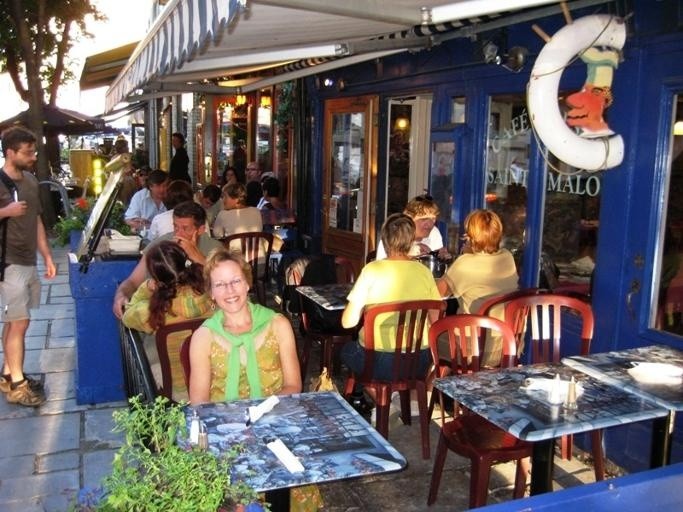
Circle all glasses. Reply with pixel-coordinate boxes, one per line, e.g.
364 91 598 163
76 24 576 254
246 168 258 171
14 149 38 156
210 275 246 290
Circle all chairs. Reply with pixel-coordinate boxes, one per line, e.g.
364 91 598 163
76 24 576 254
153 215 593 462
502 298 605 494
426 315 535 512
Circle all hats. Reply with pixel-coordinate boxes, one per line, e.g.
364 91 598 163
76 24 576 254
579 47 619 89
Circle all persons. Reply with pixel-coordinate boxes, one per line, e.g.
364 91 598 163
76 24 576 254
340 194 528 424
0 126 56 407
113 133 302 390
188 247 301 512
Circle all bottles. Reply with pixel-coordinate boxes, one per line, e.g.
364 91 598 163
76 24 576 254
564 376 578 410
549 372 562 405
198 420 209 449
189 409 199 443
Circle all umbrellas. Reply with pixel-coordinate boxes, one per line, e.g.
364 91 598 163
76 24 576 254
0 105 106 175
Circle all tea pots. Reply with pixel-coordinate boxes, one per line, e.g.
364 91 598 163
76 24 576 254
417 251 447 278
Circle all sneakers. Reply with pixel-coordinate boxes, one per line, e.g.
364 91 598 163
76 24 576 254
0 373 47 406
